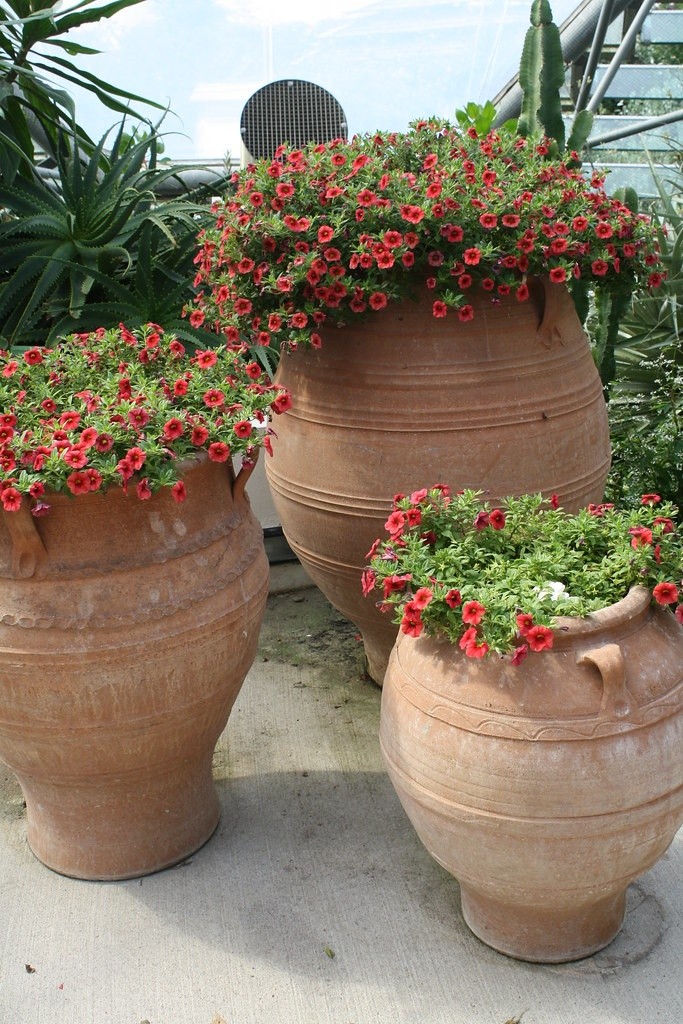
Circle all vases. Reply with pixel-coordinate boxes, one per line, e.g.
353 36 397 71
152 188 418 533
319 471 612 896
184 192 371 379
265 273 613 687
0 446 270 881
378 583 683 964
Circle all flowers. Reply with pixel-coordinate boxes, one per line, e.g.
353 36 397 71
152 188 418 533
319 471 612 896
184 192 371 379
181 117 668 356
0 321 293 519
361 479 683 669
